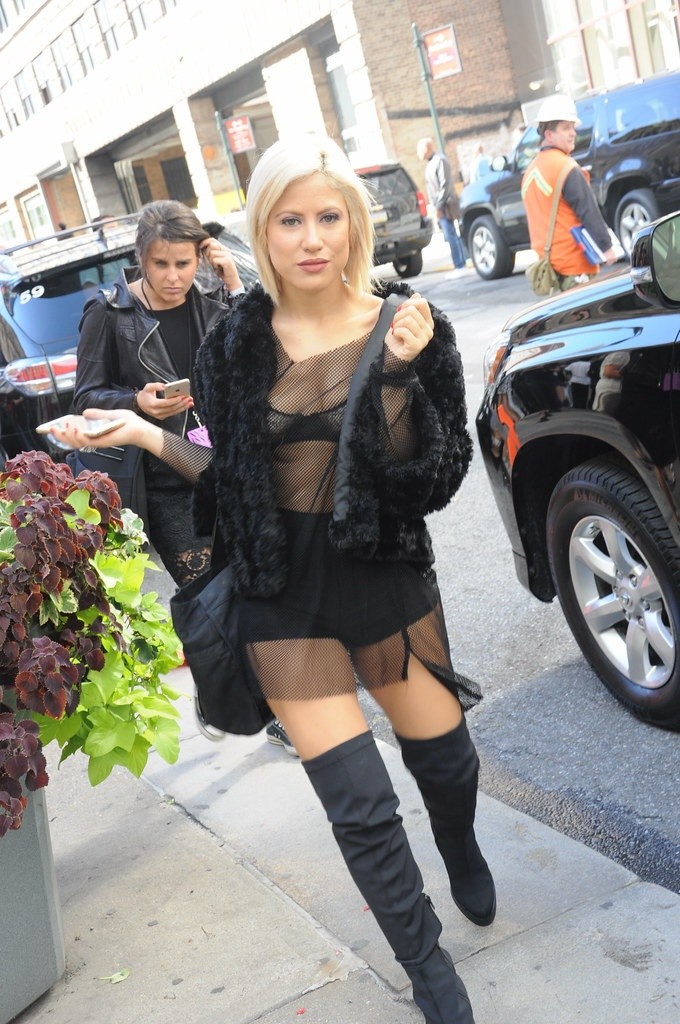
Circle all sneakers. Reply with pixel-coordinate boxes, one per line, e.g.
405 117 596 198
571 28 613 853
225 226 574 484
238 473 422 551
267 719 299 757
194 684 225 742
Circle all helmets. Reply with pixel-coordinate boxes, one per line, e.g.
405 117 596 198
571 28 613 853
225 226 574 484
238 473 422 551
537 94 582 129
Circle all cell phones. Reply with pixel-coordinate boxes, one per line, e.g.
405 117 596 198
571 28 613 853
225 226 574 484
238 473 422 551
164 378 190 400
35 414 126 439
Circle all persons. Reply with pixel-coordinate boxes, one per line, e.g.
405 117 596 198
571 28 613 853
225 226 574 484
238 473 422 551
91 215 118 234
416 138 469 281
57 223 72 241
37 134 498 1024
72 199 299 757
520 94 619 294
474 146 492 180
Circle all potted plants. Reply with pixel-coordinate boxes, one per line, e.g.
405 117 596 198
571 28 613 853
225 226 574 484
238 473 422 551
0 447 189 1024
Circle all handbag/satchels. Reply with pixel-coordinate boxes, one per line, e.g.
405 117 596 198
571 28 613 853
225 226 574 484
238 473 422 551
525 258 559 296
66 290 150 551
169 559 274 735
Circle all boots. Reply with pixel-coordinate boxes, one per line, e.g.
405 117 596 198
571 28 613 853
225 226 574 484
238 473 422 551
301 729 474 1024
393 705 497 927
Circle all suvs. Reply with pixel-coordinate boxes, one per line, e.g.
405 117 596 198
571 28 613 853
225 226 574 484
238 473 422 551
454 72 680 279
0 209 263 480
475 211 680 733
356 159 435 280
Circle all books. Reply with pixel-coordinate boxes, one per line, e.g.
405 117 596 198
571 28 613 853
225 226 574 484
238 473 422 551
581 226 626 262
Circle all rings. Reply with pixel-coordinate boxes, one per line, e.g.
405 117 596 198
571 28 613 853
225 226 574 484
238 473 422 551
79 445 98 453
